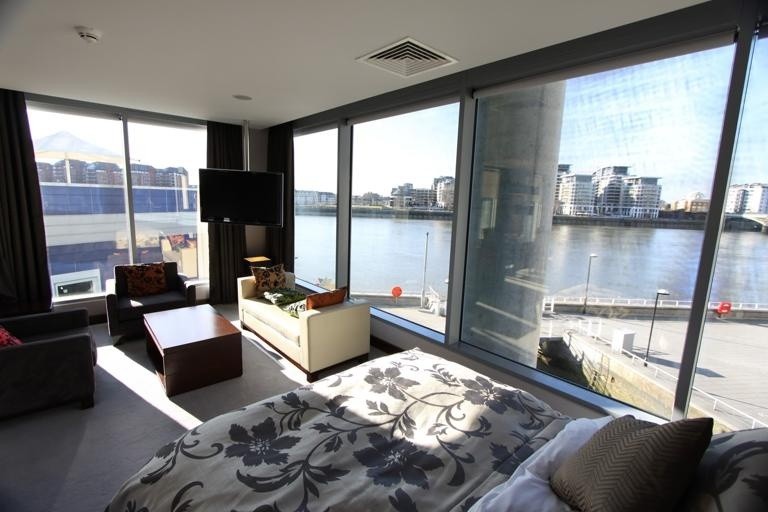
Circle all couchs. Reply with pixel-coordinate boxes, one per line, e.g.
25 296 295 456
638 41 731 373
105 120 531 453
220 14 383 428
236 272 371 382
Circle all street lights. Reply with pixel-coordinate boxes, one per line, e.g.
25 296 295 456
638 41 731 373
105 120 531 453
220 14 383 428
643 289 671 367
582 253 599 315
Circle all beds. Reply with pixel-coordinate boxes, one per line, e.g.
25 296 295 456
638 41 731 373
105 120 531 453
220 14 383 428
107 347 767 511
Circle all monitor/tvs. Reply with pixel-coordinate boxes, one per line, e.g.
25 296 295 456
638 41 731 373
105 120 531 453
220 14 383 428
199 169 284 228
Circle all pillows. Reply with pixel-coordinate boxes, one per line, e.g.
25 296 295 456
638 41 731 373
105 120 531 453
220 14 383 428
121 263 168 296
549 418 715 511
251 264 346 317
1 325 23 349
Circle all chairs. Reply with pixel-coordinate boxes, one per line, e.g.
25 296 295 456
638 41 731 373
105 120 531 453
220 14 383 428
106 263 198 347
0 308 97 420
